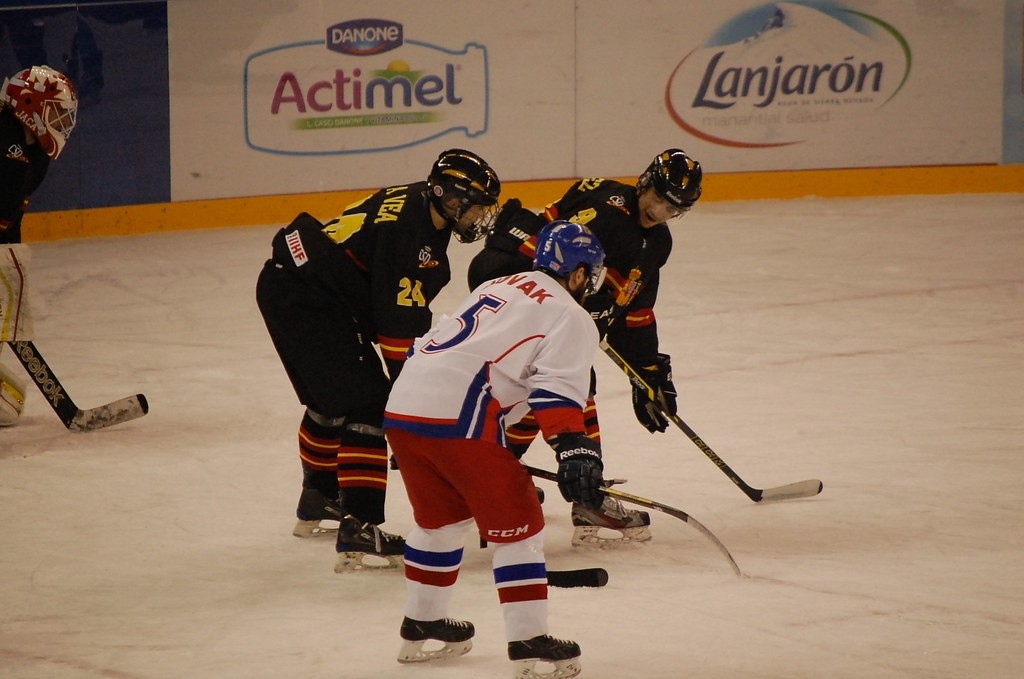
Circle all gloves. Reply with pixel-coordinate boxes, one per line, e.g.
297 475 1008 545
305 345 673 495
624 353 678 433
554 437 607 510
484 195 547 251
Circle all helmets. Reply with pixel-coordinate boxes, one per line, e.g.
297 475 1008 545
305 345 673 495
2 64 78 160
428 148 501 245
636 148 704 220
534 219 606 298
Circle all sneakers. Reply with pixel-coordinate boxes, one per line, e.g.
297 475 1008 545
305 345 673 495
333 517 407 574
571 495 652 549
292 486 341 538
397 616 475 663
508 634 583 679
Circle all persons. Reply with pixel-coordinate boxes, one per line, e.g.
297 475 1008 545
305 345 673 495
467 148 704 548
0 65 77 427
383 220 608 679
255 149 500 577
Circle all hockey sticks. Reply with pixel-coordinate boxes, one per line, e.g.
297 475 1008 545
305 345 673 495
7 340 150 433
521 464 744 579
600 340 824 505
